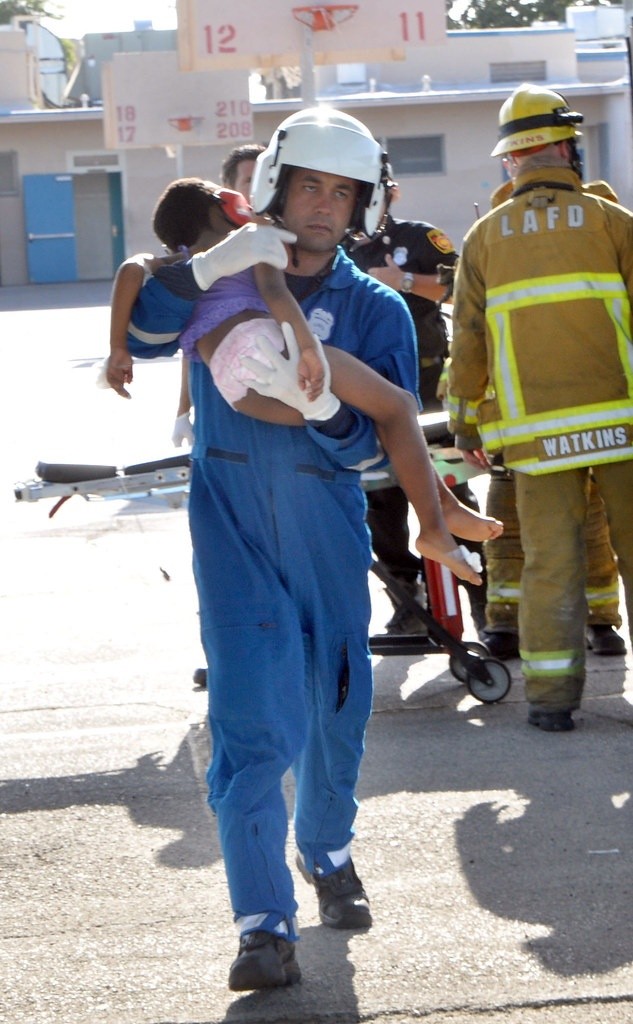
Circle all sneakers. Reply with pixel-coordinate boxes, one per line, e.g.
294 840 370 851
229 928 300 993
294 849 373 930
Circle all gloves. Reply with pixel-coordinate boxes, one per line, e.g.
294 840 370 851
239 322 341 422
191 221 296 292
170 412 194 448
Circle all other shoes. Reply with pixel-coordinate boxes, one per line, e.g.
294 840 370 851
526 700 575 732
374 630 429 658
480 633 519 662
585 621 628 656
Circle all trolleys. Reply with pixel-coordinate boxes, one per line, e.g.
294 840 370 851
14 411 513 704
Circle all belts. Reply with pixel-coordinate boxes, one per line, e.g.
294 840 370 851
419 355 444 370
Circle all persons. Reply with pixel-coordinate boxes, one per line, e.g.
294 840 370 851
172 143 627 657
449 84 633 735
107 179 504 584
125 109 422 992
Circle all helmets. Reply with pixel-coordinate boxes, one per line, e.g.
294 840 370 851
249 107 394 241
488 83 584 158
